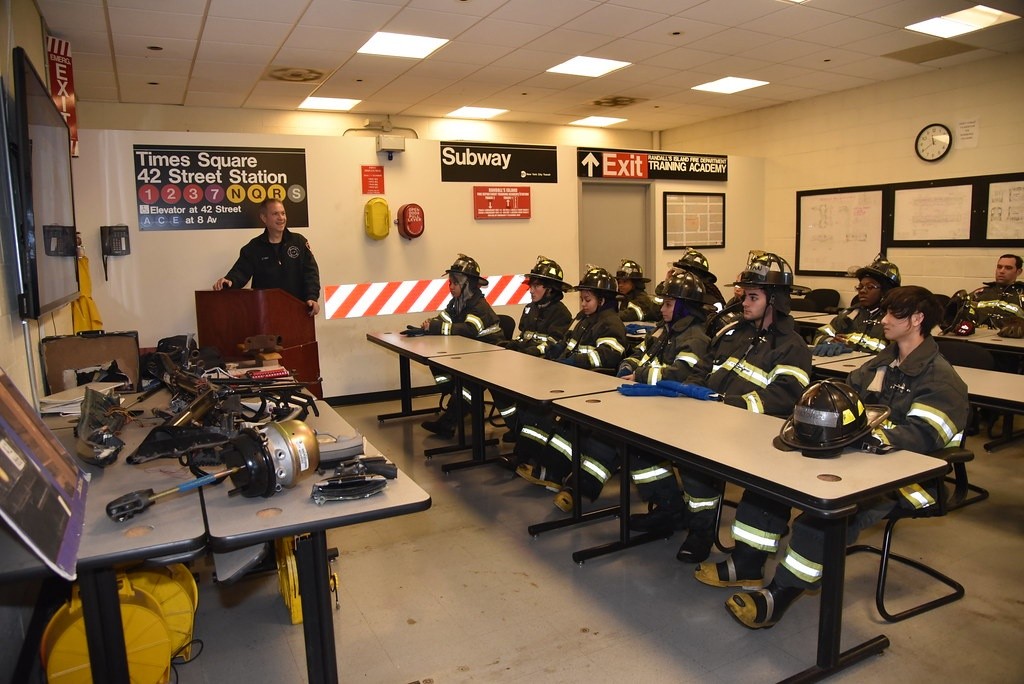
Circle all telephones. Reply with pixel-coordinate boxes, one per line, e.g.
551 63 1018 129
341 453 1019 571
100 226 130 256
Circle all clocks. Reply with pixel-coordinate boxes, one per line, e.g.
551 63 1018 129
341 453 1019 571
914 123 952 162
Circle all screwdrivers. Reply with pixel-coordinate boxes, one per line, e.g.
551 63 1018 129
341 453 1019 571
124 386 161 409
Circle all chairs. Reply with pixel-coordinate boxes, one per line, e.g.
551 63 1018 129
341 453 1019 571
845 410 989 622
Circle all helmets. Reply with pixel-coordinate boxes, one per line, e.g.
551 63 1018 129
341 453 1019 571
524 256 574 293
724 252 812 296
655 271 711 305
673 247 718 284
572 264 623 298
779 379 892 451
938 289 978 337
613 259 651 283
445 254 489 286
855 260 901 290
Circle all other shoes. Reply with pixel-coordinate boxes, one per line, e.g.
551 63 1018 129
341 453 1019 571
515 462 562 493
420 406 466 440
677 509 717 564
725 562 810 630
552 486 574 513
628 497 689 533
693 541 769 591
502 428 517 443
496 452 534 471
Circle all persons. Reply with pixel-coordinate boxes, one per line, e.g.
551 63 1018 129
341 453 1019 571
611 267 713 502
497 268 626 476
212 198 320 317
421 253 504 439
488 257 575 443
812 253 902 360
693 285 969 630
615 248 812 563
963 254 1024 437
515 267 629 513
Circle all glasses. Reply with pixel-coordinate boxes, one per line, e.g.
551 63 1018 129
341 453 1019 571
854 284 884 292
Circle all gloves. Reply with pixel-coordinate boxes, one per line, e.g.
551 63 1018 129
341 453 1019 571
617 383 684 398
399 324 427 337
810 343 853 357
656 378 723 402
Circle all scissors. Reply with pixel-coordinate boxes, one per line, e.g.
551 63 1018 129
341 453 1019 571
184 350 206 369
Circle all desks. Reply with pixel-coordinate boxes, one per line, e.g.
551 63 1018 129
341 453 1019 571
0 289 1024 684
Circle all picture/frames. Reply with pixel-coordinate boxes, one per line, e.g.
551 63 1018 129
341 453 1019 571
663 192 726 248
795 172 1024 276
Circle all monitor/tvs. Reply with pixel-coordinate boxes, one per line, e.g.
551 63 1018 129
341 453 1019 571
10 46 83 321
